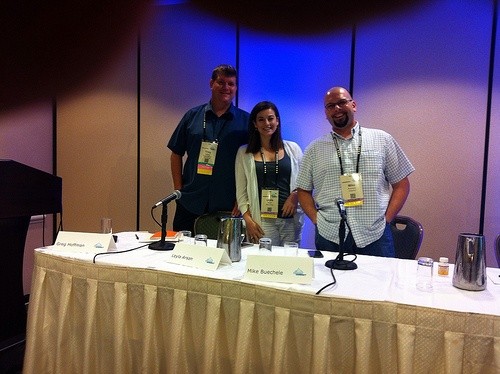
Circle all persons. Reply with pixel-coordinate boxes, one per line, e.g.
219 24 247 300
295 86 416 257
165 64 251 237
235 101 304 248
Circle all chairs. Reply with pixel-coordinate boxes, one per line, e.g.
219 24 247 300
194 211 248 242
390 215 424 260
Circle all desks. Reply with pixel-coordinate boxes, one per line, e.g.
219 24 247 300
24 231 500 374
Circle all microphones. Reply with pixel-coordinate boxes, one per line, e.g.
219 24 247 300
152 190 182 208
335 196 346 212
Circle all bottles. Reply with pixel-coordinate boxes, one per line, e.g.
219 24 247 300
438 257 449 278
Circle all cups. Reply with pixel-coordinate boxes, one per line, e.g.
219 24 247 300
284 242 298 257
259 238 271 251
416 257 433 290
194 234 207 247
179 231 191 242
100 218 112 234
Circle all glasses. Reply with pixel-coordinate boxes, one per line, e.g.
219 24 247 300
325 98 352 112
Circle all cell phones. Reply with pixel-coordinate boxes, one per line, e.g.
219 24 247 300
308 250 323 258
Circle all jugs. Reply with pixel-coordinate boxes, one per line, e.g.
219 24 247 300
453 232 487 290
217 218 241 262
